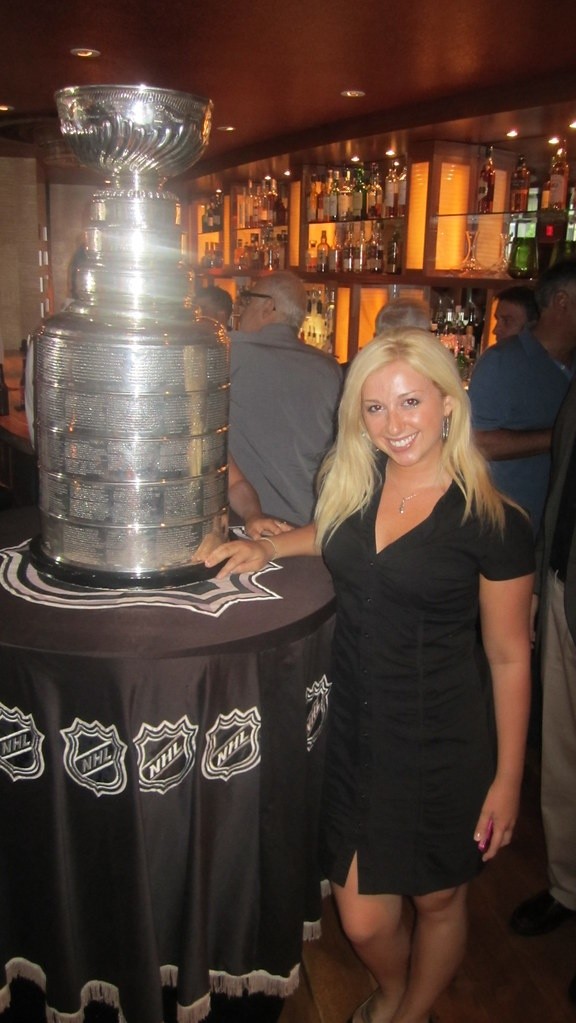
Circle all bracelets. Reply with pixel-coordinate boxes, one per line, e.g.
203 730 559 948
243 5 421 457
260 538 276 560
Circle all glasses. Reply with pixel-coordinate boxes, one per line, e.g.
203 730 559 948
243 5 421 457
239 289 276 312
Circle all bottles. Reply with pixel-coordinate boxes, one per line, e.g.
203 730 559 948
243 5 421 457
475 138 576 282
300 158 410 277
189 178 290 272
296 286 336 355
429 287 487 386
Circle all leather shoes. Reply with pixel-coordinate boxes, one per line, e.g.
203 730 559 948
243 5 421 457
509 888 576 938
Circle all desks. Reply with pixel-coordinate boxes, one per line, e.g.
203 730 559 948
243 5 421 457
0 504 335 1023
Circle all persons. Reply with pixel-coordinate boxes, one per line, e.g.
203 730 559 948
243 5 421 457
508 373 576 1010
493 287 538 342
470 255 576 770
372 298 432 336
205 326 535 1022
23 248 295 538
192 288 233 331
226 272 345 525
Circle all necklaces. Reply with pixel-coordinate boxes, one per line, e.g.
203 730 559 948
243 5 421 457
399 493 418 514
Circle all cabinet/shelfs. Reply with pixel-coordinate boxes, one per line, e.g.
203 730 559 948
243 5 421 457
166 66 576 391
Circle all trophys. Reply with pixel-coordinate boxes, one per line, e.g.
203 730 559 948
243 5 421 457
33 85 228 588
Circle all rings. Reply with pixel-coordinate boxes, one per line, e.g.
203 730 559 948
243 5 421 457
278 521 286 526
